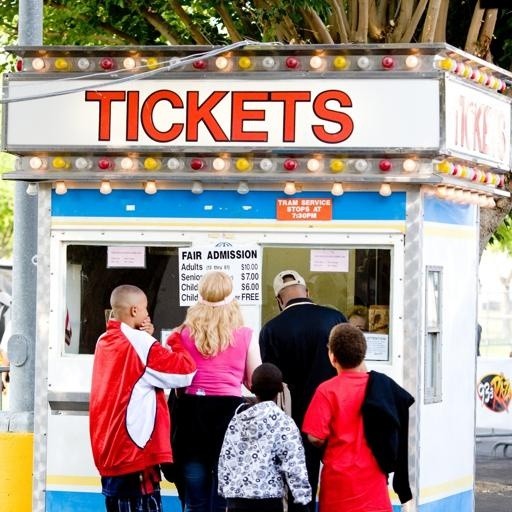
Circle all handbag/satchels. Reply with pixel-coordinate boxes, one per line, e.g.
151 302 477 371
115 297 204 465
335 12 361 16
160 389 178 483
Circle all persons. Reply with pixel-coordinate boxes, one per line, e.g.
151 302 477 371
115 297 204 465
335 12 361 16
216 362 314 512
258 270 349 511
345 304 369 334
168 270 258 512
93 284 198 511
299 322 395 510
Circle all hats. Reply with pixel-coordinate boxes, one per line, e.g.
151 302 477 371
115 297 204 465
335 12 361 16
274 270 306 311
